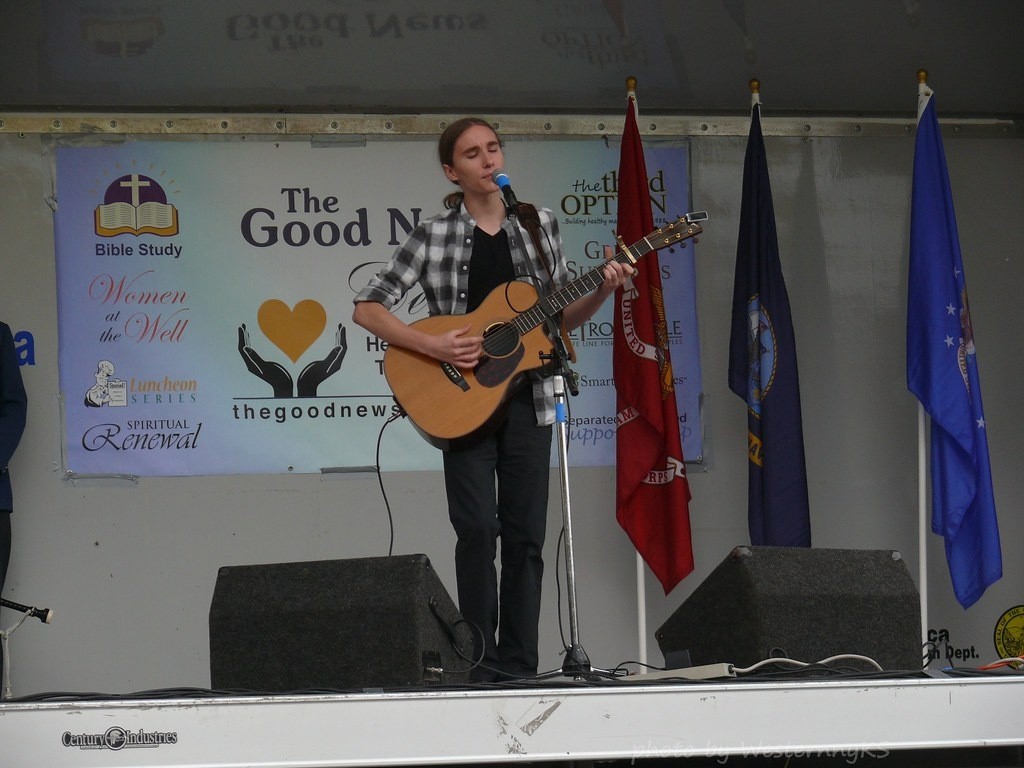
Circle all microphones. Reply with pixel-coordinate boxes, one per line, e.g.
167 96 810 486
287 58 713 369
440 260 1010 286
491 167 520 217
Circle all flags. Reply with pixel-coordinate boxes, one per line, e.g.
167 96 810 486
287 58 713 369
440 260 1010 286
907 91 1004 610
729 102 812 548
614 98 695 596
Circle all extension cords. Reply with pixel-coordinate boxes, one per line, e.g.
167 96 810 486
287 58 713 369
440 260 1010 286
614 663 737 681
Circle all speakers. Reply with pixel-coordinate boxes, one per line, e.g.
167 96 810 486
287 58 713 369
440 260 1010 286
210 553 473 689
654 545 921 681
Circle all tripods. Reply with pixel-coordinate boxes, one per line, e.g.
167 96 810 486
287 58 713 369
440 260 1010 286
505 202 627 683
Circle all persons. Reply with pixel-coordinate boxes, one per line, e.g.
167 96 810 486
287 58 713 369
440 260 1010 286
351 117 634 685
0 319 28 698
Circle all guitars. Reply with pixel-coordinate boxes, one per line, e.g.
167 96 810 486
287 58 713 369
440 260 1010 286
383 210 709 453
